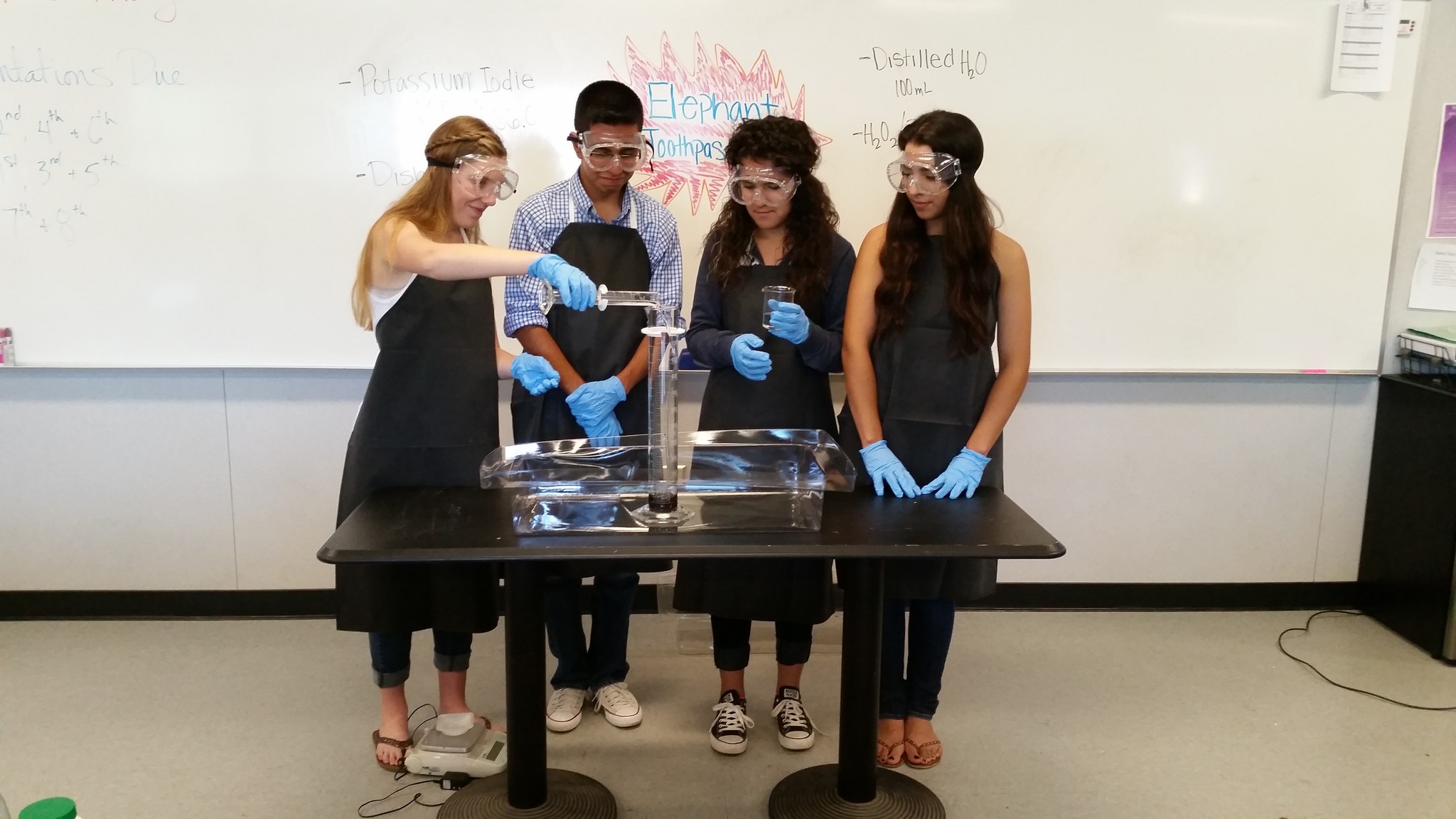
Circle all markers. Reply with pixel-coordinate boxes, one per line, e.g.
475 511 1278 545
0 328 12 363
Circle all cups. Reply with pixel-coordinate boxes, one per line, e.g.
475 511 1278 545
761 285 796 331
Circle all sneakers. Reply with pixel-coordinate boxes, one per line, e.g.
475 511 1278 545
546 687 588 731
593 681 642 728
772 685 829 750
709 688 754 754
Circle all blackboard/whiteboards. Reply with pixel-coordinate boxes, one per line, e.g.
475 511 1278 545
0 0 1430 377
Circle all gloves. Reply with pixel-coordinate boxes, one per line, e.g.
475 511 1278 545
511 352 561 396
767 299 810 345
565 376 626 426
859 440 921 498
921 446 992 500
527 253 597 311
583 411 623 453
729 333 772 380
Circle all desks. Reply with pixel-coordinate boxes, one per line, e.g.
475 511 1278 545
1354 372 1456 667
315 484 1066 819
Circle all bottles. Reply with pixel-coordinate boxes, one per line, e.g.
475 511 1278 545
18 796 82 819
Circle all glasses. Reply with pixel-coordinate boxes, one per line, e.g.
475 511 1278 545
453 154 519 200
577 131 647 171
727 164 801 207
887 152 960 195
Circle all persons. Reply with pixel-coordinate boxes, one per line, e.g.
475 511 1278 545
840 113 1032 766
670 117 857 757
333 115 595 771
505 81 682 730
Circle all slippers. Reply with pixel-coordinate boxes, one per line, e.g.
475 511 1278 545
480 716 507 734
877 738 906 768
372 729 414 773
904 737 941 769
